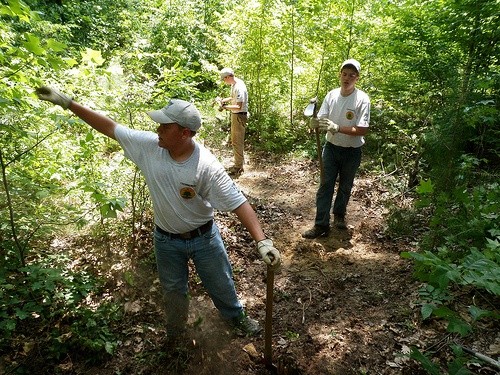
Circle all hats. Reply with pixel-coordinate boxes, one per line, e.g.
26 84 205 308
146 100 202 131
218 68 234 80
341 59 362 74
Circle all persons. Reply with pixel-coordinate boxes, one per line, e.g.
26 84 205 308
217 66 248 175
36 85 280 352
301 57 370 240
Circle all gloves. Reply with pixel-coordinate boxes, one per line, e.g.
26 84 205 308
35 84 73 110
310 116 319 129
319 118 339 135
256 239 282 271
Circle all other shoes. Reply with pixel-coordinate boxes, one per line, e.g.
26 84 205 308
302 225 331 239
158 335 184 369
234 315 263 336
334 216 348 230
225 166 243 175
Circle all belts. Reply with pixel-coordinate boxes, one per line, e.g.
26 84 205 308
155 220 213 240
233 112 247 114
